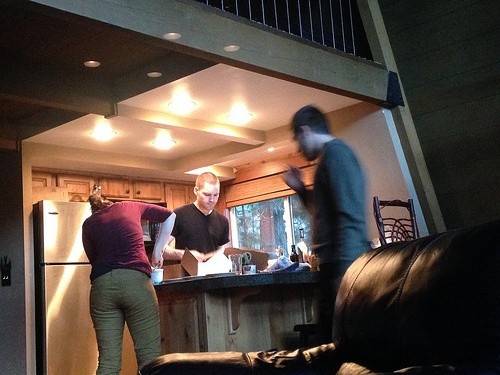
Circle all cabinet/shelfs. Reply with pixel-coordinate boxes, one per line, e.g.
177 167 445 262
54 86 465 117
98 174 166 204
32 171 98 202
164 179 217 213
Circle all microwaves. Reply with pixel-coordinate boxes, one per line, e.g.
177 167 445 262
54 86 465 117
140 219 162 253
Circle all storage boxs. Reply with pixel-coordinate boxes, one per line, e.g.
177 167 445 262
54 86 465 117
161 247 232 280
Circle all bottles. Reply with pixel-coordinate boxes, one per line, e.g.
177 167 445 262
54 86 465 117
276 246 284 258
290 245 299 263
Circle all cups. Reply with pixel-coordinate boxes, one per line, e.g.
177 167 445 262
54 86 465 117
228 254 243 275
243 264 256 274
152 268 164 284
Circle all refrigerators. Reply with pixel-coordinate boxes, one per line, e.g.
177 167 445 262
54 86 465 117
33 199 138 375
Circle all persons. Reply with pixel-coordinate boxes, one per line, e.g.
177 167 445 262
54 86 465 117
162 172 231 266
283 104 370 345
82 193 176 375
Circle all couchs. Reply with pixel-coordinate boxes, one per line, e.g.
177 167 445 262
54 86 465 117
137 218 500 375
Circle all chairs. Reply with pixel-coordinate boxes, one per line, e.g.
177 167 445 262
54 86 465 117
293 195 420 347
